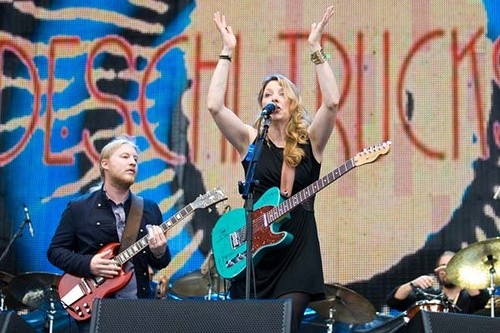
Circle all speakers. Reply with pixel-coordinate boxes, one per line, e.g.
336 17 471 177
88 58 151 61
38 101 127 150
392 309 500 332
89 296 297 333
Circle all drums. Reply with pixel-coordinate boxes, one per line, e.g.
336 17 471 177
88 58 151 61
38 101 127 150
406 299 450 320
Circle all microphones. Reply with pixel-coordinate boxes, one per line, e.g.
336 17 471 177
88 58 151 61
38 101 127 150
23 205 37 237
262 102 277 116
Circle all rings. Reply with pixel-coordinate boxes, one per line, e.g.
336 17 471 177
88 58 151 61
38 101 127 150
153 242 156 243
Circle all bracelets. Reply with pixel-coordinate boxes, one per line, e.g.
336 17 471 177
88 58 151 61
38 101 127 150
311 49 327 64
219 55 231 62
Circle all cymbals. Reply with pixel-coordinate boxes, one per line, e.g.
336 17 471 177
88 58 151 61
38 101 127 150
9 271 64 312
308 283 376 324
444 237 500 289
172 268 232 297
0 270 15 301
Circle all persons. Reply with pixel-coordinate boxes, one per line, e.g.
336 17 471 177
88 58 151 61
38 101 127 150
387 250 500 317
206 4 340 333
47 140 171 333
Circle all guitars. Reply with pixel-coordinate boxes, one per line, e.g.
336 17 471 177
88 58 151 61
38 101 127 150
211 139 392 280
58 184 227 322
